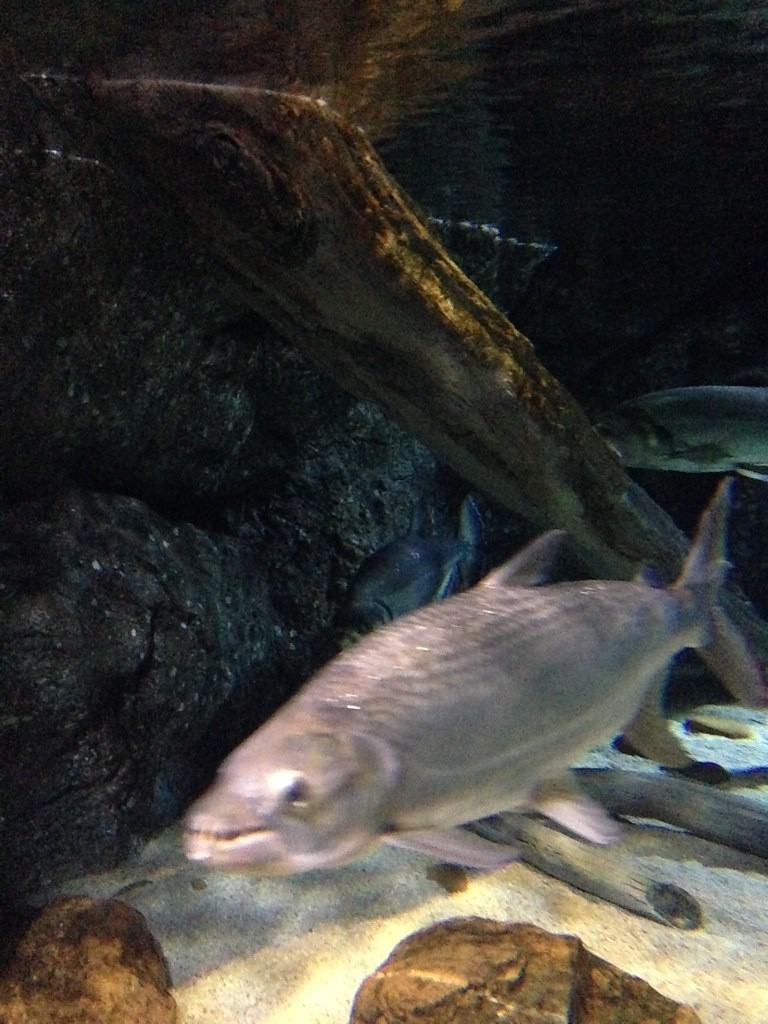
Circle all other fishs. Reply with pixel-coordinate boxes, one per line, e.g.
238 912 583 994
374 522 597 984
175 476 768 880
594 385 768 484
